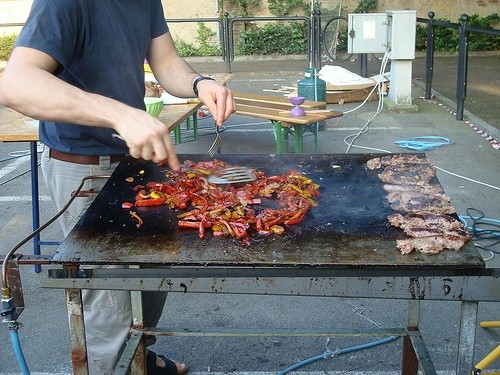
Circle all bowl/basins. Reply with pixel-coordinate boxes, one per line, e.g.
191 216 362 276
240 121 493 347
143 97 163 117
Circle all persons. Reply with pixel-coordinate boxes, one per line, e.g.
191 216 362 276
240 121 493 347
0 0 236 375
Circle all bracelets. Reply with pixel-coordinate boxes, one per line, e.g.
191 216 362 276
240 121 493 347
193 77 214 95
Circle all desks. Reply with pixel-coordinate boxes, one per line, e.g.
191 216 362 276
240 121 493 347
0 72 236 273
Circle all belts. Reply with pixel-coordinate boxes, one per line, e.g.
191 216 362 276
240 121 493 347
50 148 125 165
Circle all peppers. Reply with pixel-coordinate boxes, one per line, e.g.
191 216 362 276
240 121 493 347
123 159 320 239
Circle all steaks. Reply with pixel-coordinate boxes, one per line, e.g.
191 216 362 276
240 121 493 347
366 154 473 255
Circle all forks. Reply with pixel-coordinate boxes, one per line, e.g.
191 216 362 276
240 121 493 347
208 123 221 157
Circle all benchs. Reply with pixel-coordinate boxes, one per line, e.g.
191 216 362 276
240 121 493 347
173 91 344 154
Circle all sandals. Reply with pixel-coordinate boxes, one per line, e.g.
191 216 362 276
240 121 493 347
146 349 188 375
